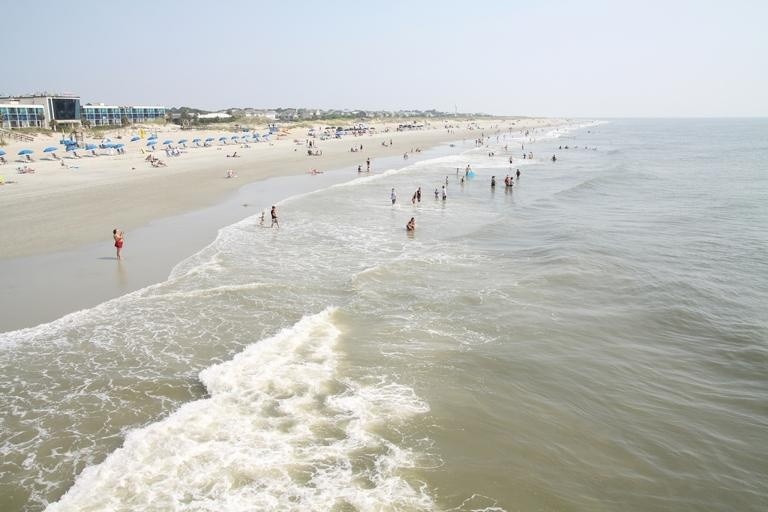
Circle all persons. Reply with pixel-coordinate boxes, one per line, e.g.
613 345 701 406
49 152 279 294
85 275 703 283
114 228 123 255
0 118 597 233
